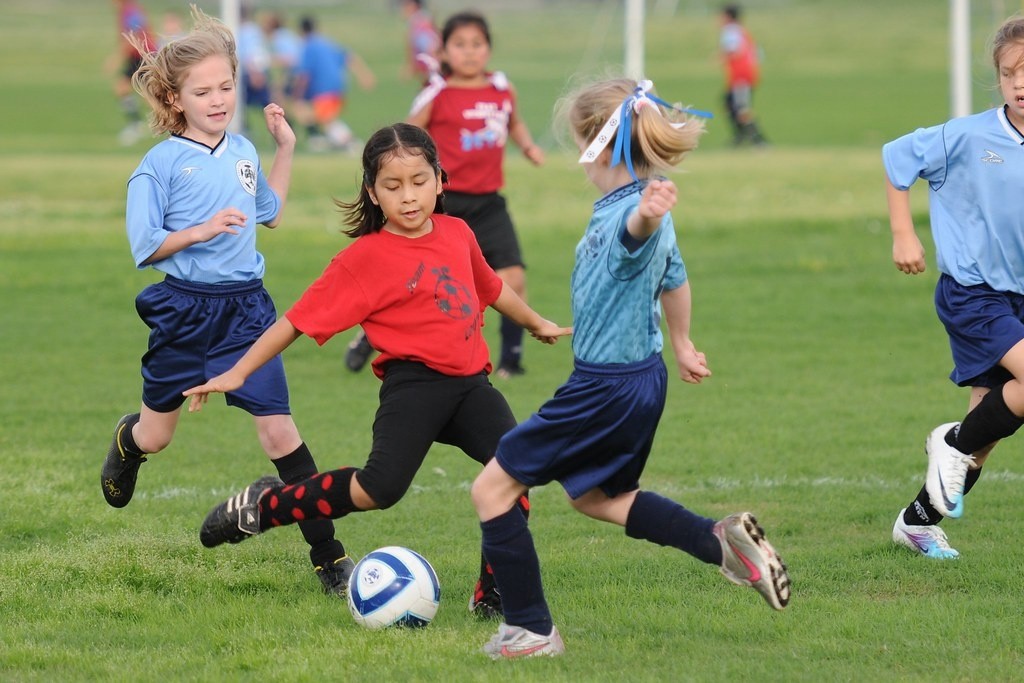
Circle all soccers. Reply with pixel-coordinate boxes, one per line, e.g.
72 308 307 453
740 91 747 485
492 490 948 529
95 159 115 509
347 545 441 630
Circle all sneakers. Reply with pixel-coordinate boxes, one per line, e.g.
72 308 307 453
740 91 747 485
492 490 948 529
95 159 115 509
496 361 526 378
100 413 145 508
468 622 565 662
309 541 354 599
713 513 793 612
469 580 503 617
347 329 374 371
925 423 978 521
891 508 960 561
199 476 284 548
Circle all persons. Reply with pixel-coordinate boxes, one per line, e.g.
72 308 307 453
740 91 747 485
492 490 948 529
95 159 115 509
239 2 376 154
720 6 766 143
100 16 357 599
882 15 1024 560
471 78 792 659
400 0 446 89
347 12 544 379
182 123 574 619
107 0 186 143
